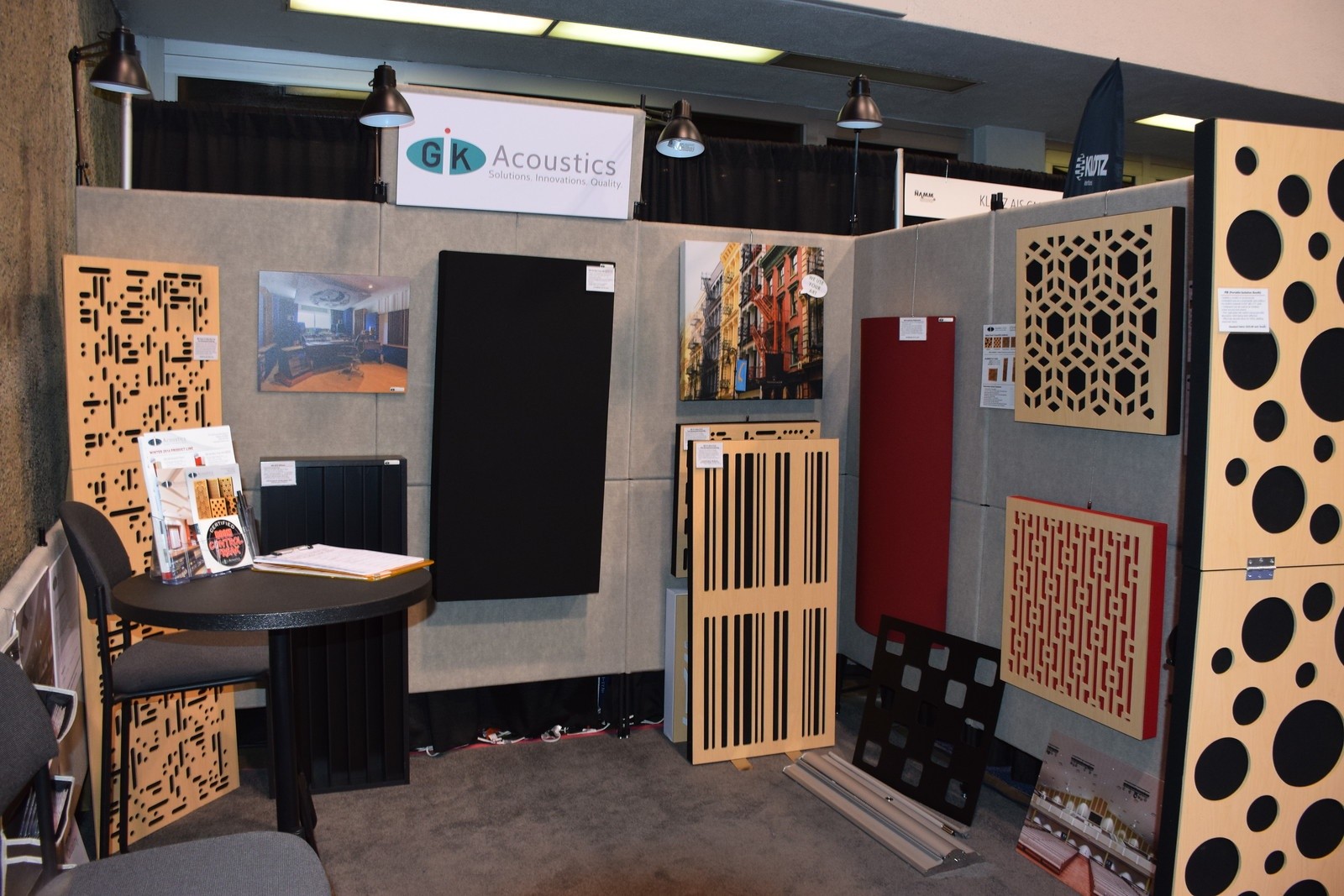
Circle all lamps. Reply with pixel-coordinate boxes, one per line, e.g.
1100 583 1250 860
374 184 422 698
66 23 153 188
783 749 984 876
359 60 414 205
642 94 706 160
836 74 882 238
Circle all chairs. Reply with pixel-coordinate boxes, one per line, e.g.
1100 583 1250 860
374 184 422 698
336 334 372 378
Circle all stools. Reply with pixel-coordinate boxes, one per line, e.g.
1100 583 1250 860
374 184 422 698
58 498 321 864
0 650 332 896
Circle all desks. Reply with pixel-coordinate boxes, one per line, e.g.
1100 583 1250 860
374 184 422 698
110 560 431 847
